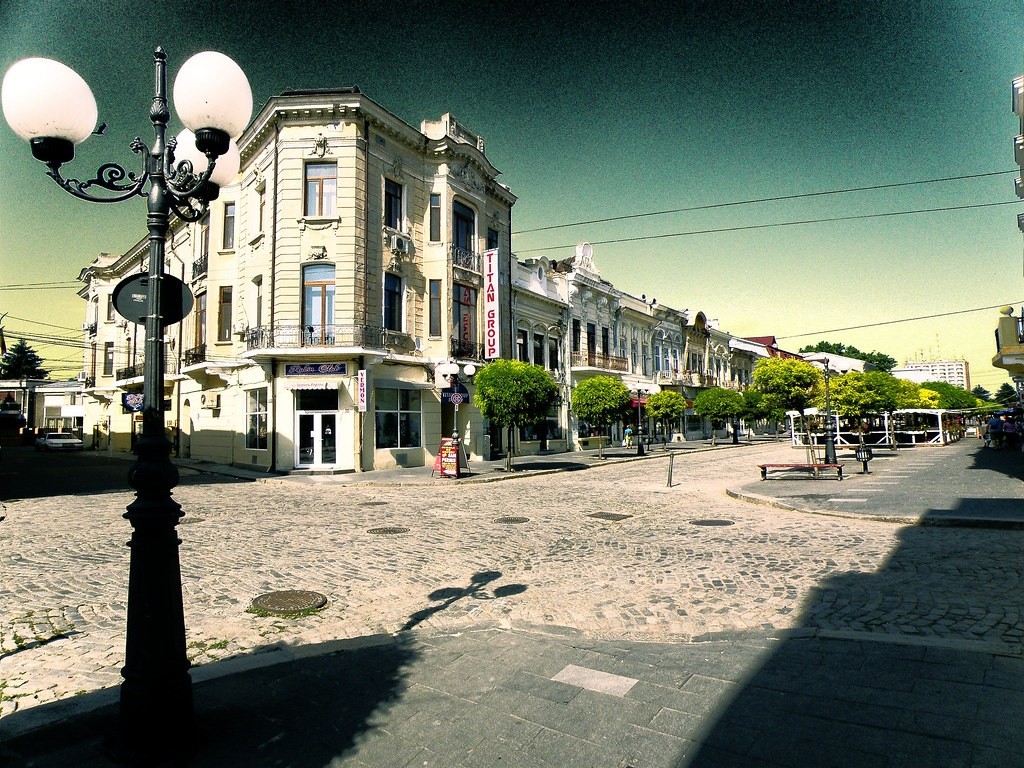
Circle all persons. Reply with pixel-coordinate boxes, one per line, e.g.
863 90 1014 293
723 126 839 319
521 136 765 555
624 425 634 450
959 413 1024 454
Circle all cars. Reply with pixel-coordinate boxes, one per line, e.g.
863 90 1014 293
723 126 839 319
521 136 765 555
36 432 85 452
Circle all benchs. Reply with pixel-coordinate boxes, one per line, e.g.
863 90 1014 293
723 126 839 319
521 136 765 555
759 463 845 481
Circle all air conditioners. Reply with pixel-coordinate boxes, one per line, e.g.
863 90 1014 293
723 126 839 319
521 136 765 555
391 235 409 254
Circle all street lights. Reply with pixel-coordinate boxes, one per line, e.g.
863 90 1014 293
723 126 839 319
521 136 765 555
628 379 659 456
0 43 258 768
823 355 839 463
434 361 476 478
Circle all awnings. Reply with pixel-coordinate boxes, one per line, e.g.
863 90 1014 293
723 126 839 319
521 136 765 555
629 394 646 408
372 377 436 391
287 379 343 390
